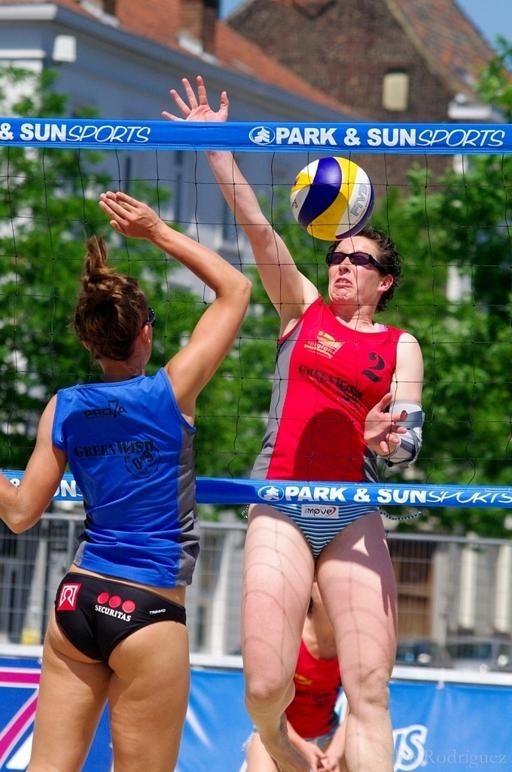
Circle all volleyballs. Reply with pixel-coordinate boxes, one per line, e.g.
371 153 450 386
290 157 374 241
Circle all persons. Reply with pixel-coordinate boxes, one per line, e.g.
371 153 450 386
163 76 423 772
245 563 352 772
0 191 252 772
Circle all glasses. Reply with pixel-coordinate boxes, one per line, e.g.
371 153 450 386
142 307 156 333
325 252 387 277
